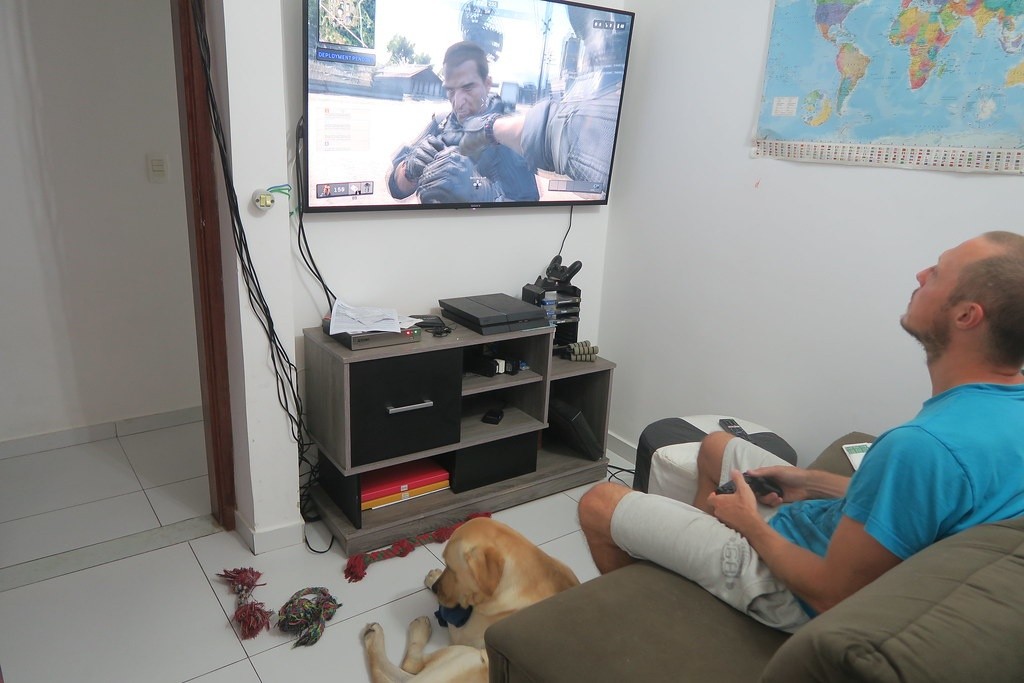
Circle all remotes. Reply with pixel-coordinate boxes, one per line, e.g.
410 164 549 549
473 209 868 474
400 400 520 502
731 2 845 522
718 418 757 445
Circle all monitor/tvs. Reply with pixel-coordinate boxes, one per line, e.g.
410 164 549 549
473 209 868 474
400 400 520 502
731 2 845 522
303 0 636 213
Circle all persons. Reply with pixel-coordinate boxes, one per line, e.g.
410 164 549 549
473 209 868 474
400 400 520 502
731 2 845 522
578 229 1024 630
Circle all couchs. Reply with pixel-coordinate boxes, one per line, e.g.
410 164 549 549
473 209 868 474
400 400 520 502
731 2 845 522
484 433 1024 683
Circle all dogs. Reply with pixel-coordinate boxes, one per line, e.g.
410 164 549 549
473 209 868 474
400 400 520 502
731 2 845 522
359 517 580 683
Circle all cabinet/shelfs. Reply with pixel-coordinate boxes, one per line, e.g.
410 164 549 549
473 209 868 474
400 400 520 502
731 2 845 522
302 309 618 555
522 282 582 349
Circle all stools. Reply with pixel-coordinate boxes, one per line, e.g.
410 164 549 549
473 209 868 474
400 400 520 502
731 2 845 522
632 415 799 506
